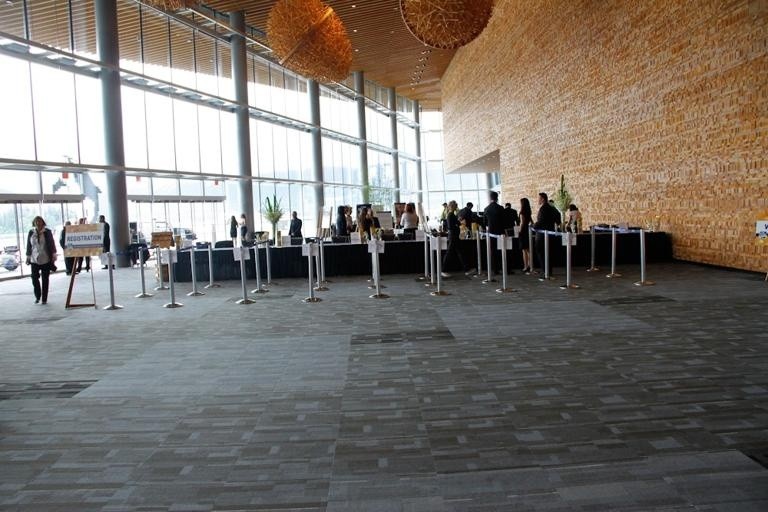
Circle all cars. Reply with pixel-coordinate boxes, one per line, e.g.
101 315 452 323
173 228 196 243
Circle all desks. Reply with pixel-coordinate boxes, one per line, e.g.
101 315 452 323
173 231 673 288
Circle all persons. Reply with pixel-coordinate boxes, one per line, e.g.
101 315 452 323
25 216 57 305
239 214 248 239
59 220 78 276
98 214 116 270
288 210 303 245
76 218 90 273
230 215 239 247
335 191 583 278
